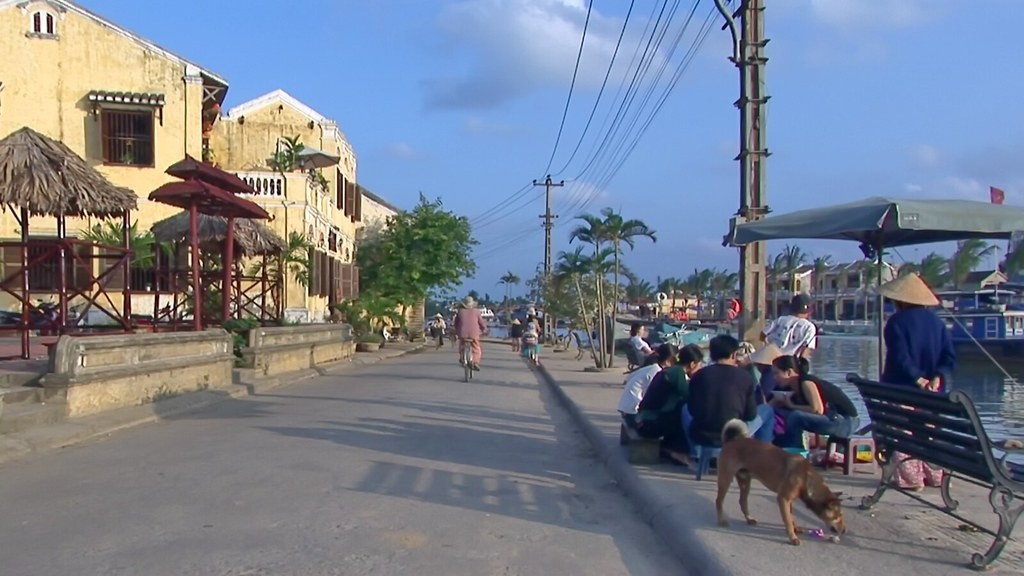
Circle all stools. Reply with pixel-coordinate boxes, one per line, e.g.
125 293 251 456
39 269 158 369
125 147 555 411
824 435 877 480
696 447 720 481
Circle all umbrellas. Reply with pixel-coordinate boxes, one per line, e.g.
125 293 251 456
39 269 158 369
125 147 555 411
721 196 1024 382
264 143 341 172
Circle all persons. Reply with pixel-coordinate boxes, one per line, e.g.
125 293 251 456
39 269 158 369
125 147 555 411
434 312 446 345
522 307 541 366
455 296 487 371
630 323 655 366
760 294 818 356
511 319 522 351
618 332 859 473
875 272 956 492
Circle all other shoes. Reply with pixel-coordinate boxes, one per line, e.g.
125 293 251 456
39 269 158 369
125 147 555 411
665 449 698 465
474 362 480 369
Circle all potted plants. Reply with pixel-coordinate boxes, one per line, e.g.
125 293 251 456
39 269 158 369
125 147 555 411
359 335 383 352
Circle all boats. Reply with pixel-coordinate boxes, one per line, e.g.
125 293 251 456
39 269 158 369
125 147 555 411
932 290 1024 352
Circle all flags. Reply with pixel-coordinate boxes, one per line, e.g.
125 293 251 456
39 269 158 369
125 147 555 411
990 187 1005 203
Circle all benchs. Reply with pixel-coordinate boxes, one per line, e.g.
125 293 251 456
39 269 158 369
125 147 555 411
546 331 596 360
617 417 664 464
846 371 1023 569
620 338 659 374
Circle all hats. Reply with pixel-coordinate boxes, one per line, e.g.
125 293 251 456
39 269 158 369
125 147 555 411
527 307 536 316
435 313 442 318
513 319 521 325
876 272 941 307
460 296 478 308
790 294 813 314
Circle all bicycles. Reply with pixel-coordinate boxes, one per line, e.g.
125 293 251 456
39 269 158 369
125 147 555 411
526 345 536 365
433 335 440 350
461 340 478 382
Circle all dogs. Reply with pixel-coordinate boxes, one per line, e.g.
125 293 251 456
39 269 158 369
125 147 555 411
716 417 848 546
